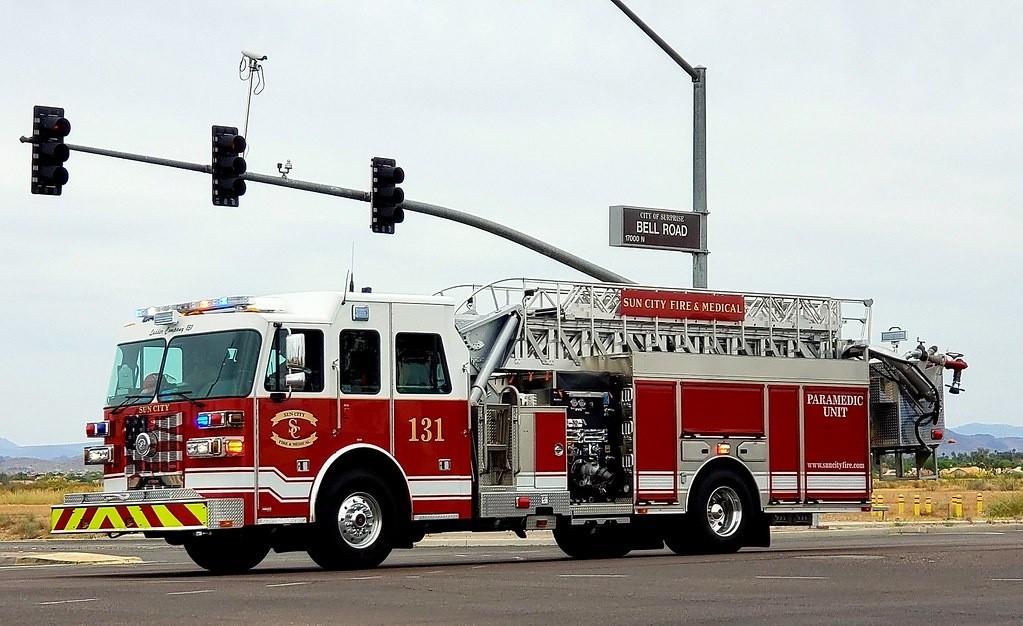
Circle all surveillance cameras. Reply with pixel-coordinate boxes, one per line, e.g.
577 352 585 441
241 50 268 62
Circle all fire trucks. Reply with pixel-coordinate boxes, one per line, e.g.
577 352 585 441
49 293 969 573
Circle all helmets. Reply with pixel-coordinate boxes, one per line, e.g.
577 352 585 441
141 373 168 394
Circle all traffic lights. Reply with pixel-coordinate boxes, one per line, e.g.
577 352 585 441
31 105 70 197
370 157 405 235
212 125 247 209
